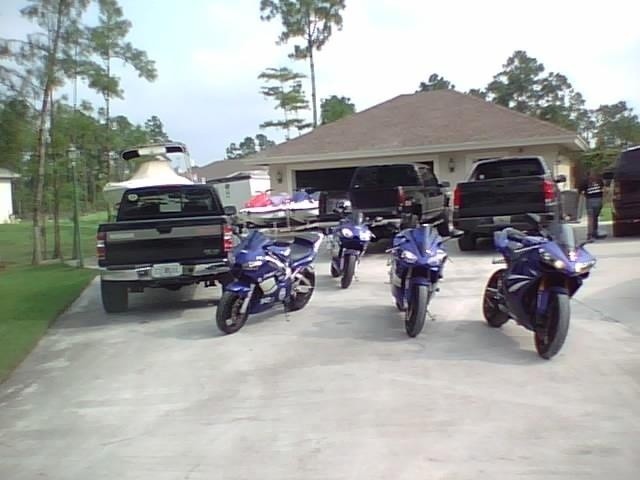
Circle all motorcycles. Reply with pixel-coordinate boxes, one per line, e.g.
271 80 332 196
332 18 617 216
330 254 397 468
216 225 324 334
384 221 464 338
483 213 608 360
331 207 383 289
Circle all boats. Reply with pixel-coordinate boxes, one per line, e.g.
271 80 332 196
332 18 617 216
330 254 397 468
238 201 320 225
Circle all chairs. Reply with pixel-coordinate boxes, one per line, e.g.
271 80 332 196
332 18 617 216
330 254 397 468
141 201 208 216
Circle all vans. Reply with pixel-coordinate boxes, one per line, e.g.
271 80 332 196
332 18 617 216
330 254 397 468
604 146 640 237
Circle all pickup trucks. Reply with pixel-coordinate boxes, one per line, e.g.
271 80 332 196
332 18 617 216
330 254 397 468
96 184 236 313
452 155 566 251
317 163 454 242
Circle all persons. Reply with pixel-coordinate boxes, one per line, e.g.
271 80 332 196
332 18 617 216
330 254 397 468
581 167 609 238
